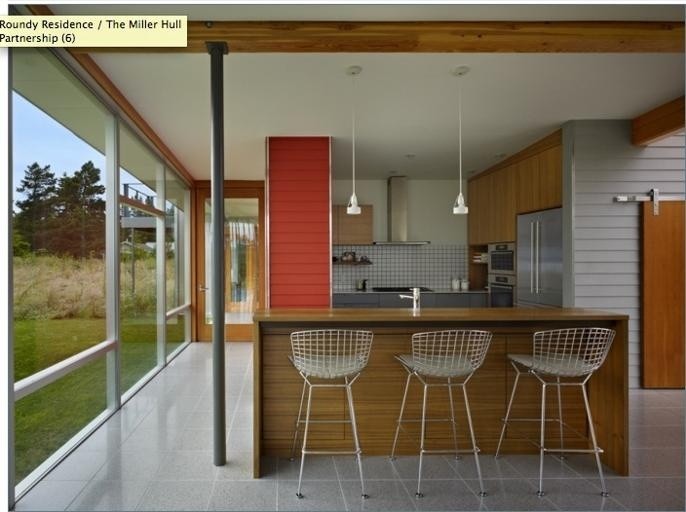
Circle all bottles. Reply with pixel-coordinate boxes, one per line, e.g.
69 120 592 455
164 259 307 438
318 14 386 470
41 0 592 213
452 279 469 290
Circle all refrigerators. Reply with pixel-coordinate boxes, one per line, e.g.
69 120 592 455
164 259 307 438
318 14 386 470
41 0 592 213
513 208 563 308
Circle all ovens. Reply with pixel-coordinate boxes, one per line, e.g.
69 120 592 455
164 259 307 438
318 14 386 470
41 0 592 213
488 242 517 307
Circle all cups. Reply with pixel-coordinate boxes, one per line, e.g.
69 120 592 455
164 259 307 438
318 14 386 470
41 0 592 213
360 256 368 261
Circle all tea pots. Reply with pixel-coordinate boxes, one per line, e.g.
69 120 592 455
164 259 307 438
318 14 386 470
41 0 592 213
340 251 355 262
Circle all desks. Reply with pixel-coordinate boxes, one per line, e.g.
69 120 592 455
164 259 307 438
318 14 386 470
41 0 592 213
253 308 631 477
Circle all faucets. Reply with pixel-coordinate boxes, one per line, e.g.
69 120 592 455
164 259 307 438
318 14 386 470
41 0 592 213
399 286 420 308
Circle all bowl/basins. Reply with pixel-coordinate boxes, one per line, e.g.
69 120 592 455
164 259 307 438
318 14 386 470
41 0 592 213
471 252 487 262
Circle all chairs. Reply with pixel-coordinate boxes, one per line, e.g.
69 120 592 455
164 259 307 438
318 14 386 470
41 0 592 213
390 329 492 499
287 329 374 499
494 327 617 497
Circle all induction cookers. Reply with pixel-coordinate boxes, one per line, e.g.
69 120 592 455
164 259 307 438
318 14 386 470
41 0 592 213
372 287 434 292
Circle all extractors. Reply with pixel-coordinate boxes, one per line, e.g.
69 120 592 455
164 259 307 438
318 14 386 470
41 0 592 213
373 176 431 245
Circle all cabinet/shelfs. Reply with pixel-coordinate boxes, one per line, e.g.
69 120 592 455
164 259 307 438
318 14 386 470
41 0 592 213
466 130 563 291
332 205 374 245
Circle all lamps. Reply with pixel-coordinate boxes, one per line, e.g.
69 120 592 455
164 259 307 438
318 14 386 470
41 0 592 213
346 66 362 215
453 66 468 216
